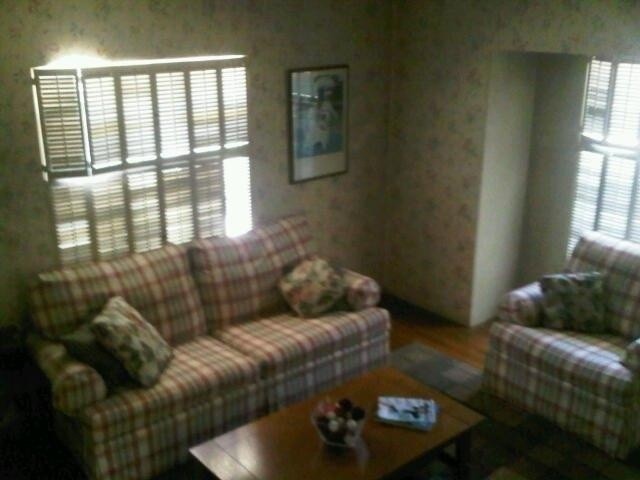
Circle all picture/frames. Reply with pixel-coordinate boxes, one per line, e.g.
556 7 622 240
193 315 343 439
288 64 349 184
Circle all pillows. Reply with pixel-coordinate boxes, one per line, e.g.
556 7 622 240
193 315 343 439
538 230 640 339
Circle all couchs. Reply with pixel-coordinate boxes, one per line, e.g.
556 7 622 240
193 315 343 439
24 216 392 480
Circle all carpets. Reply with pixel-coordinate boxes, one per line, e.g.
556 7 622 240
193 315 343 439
385 340 639 479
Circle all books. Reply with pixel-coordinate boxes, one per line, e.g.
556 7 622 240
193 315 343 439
373 397 437 433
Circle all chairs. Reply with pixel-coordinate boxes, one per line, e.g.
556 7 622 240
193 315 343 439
481 230 640 461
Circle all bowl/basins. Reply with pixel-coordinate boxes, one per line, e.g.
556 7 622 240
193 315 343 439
311 396 368 447
